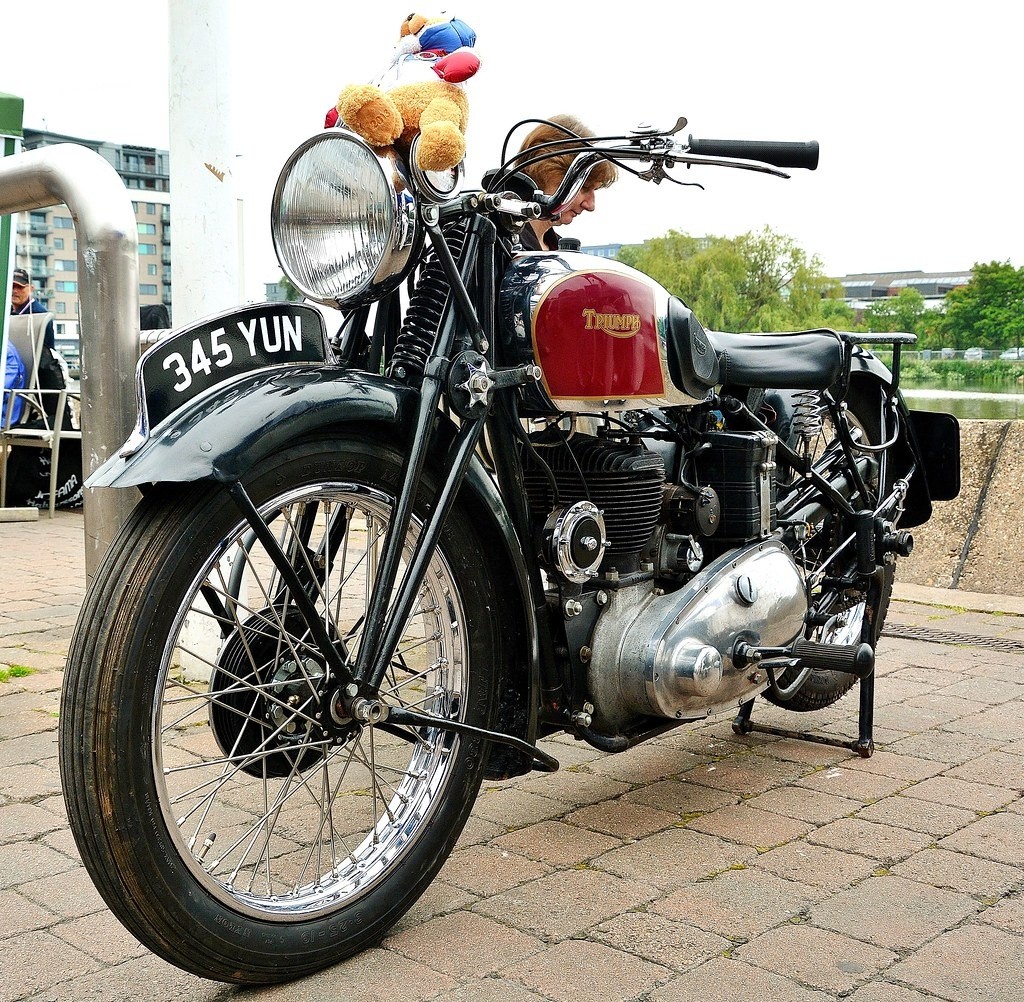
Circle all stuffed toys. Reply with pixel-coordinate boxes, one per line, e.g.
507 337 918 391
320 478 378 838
334 6 482 172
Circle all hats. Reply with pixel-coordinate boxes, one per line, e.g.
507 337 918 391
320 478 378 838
10 268 30 287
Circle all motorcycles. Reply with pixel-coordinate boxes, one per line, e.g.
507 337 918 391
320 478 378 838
57 116 961 990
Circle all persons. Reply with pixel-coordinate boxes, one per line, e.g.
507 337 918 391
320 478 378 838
511 114 619 254
0 269 55 430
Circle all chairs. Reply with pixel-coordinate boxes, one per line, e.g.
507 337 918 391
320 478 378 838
0 389 81 519
0 311 54 446
